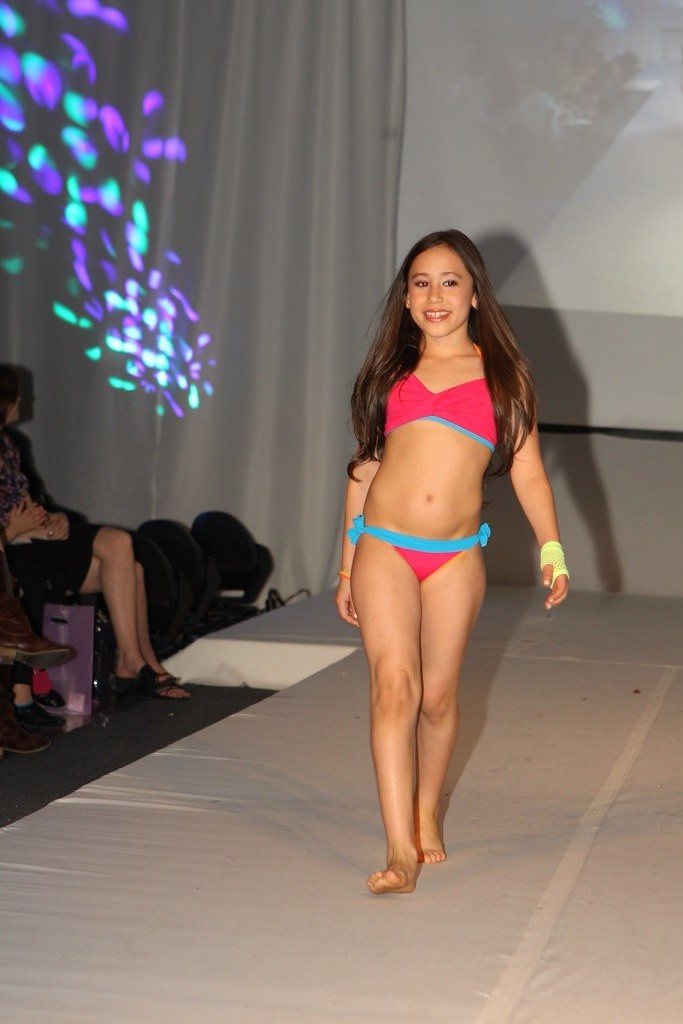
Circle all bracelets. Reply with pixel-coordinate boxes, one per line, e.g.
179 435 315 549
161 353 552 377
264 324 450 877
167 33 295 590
337 570 350 582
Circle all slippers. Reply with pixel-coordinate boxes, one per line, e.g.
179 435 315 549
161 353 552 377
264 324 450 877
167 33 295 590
146 685 192 701
115 664 177 706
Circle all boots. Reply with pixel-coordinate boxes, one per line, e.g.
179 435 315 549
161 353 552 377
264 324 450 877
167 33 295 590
0 594 71 668
1 687 52 758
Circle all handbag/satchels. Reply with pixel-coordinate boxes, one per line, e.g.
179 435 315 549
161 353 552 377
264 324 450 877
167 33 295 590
43 602 94 716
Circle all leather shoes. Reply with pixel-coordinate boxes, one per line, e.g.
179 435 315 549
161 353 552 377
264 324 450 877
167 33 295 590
16 704 65 731
33 668 67 708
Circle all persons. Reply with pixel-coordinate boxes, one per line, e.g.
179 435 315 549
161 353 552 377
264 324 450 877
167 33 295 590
9 547 66 734
333 228 578 896
0 361 192 709
1 497 69 707
0 548 76 762
